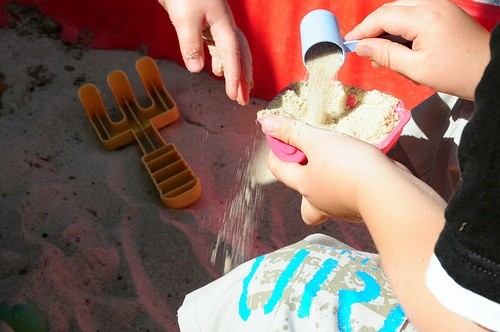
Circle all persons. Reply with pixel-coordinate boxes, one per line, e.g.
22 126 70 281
260 0 500 332
158 0 254 107
